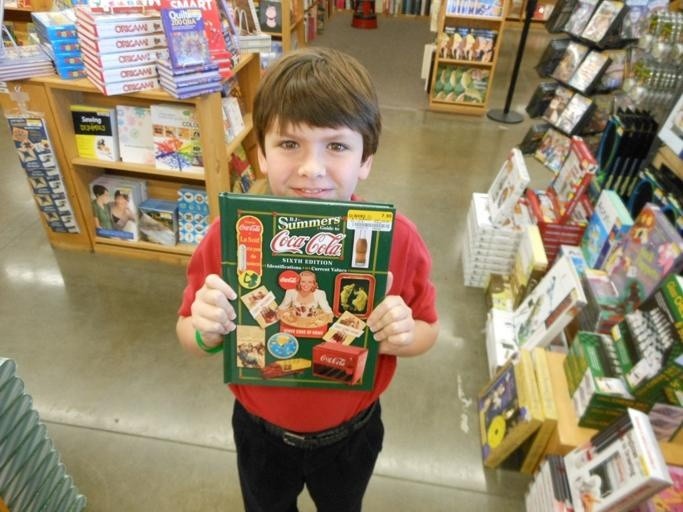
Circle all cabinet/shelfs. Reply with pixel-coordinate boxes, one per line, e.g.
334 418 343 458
427 0 510 117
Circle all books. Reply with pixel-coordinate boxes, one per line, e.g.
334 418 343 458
1 0 337 510
383 0 432 17
433 0 682 510
217 192 396 392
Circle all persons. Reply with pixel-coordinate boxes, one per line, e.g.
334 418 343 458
176 46 441 510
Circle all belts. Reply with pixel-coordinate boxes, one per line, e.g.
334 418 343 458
242 400 377 448
